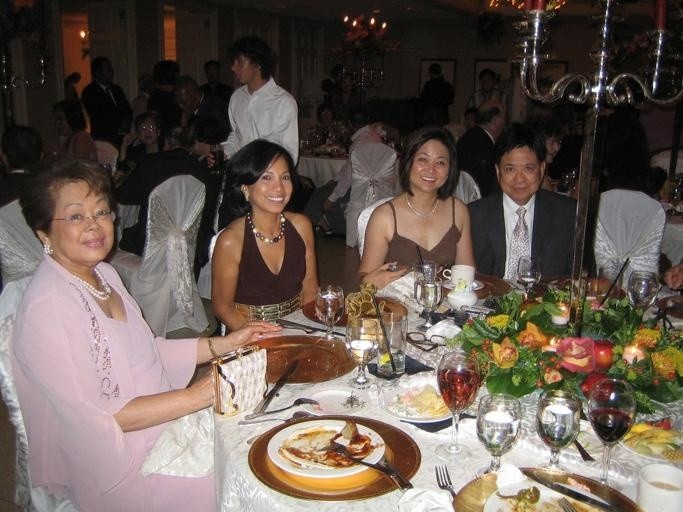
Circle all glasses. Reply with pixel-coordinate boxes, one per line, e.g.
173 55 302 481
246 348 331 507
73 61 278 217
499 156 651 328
139 122 156 134
45 209 112 226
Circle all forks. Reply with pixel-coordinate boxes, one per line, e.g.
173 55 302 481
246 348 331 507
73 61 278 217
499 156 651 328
317 444 398 477
556 496 572 506
434 465 458 500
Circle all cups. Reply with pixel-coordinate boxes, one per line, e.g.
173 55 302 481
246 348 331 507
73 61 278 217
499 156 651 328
588 378 638 485
209 143 225 176
413 262 437 284
638 463 683 507
344 318 381 390
565 165 578 180
314 285 345 344
375 312 407 377
516 256 542 302
628 270 659 311
415 279 445 331
437 350 482 463
474 392 524 477
443 264 477 287
534 390 582 467
596 267 624 310
249 304 278 328
556 169 570 193
668 183 680 217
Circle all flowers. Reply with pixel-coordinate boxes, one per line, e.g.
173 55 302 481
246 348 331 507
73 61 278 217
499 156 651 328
449 256 683 420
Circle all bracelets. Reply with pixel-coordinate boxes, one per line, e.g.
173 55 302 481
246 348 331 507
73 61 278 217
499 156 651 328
208 337 219 359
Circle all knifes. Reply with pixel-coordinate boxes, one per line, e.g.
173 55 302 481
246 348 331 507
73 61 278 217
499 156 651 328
519 468 615 508
275 317 344 337
379 455 415 494
251 358 303 415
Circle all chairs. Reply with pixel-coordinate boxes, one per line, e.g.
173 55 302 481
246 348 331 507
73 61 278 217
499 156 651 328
596 188 668 280
346 140 398 249
95 140 120 175
110 174 206 338
0 198 46 290
0 276 33 512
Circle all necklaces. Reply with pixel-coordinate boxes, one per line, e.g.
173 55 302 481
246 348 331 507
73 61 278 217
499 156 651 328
73 268 111 300
245 207 286 244
405 190 439 218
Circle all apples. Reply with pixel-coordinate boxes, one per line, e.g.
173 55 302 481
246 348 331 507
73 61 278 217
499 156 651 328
594 338 614 369
580 372 612 402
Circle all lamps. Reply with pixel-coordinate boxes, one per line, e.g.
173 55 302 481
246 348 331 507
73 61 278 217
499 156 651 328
488 2 682 143
79 29 91 61
339 9 393 90
0 1 47 93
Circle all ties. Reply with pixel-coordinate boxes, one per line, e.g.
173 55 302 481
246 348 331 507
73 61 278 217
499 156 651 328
509 207 531 279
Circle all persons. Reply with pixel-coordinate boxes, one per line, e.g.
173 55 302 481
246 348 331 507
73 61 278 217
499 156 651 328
456 100 507 177
147 61 181 127
133 74 153 116
42 99 97 161
329 72 363 116
467 68 503 110
12 156 283 512
64 72 86 132
539 120 564 192
302 112 402 238
356 127 477 289
467 123 578 279
172 75 231 145
413 63 455 128
0 125 45 209
117 126 198 255
199 60 233 143
207 36 299 169
116 112 162 172
464 106 481 128
83 57 134 148
210 139 320 331
185 114 222 160
315 104 353 145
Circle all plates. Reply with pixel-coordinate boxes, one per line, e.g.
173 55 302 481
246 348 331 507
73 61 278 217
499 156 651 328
656 294 683 319
300 387 371 416
266 418 386 479
547 279 617 300
437 280 484 292
618 438 667 462
240 334 360 385
452 466 639 508
437 271 512 300
247 413 423 503
379 371 452 424
302 295 408 328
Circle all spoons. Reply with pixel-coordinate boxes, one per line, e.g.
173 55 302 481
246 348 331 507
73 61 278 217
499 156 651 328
274 321 322 335
246 410 314 446
243 396 321 421
